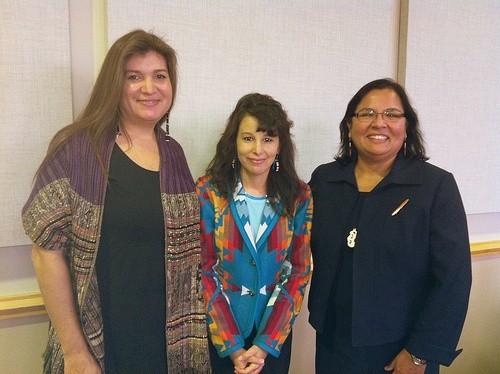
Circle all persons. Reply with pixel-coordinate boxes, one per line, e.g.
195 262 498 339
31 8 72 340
192 91 320 374
308 77 475 374
20 29 218 372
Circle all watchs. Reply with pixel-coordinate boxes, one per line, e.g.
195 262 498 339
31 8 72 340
409 354 427 366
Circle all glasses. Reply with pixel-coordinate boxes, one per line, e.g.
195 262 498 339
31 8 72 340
351 108 406 121
